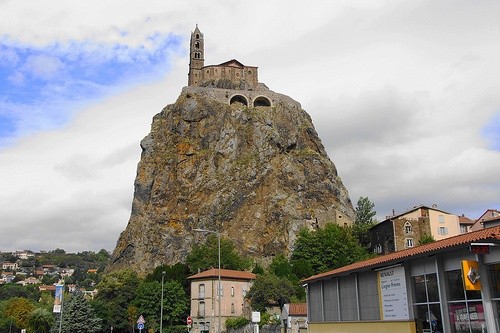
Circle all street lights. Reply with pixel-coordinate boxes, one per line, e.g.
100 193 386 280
192 228 222 333
160 271 166 333
